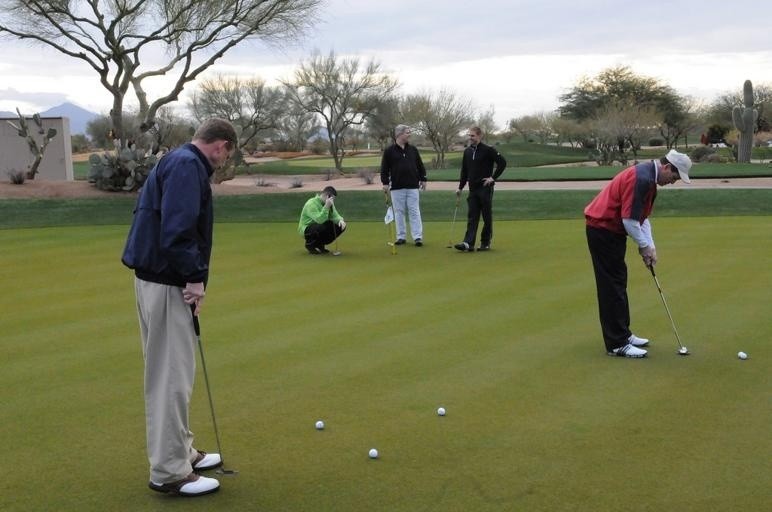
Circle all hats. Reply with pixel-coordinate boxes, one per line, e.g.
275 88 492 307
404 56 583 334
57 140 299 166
666 149 692 184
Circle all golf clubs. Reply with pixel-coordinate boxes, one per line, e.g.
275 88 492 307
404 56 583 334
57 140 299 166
386 198 398 255
653 261 689 355
329 199 342 254
191 303 237 475
445 193 460 249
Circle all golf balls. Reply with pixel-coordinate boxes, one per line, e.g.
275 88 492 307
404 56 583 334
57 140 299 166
368 447 379 459
438 408 444 417
680 347 686 353
738 350 745 362
315 422 323 429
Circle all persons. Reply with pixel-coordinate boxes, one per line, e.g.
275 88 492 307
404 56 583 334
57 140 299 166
381 124 426 247
297 186 346 255
121 119 237 497
584 149 692 358
454 126 506 252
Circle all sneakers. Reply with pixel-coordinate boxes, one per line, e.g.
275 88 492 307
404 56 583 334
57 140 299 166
455 242 474 250
607 334 649 357
305 243 329 255
149 472 220 497
395 238 406 245
477 245 490 250
190 450 222 471
414 239 423 246
